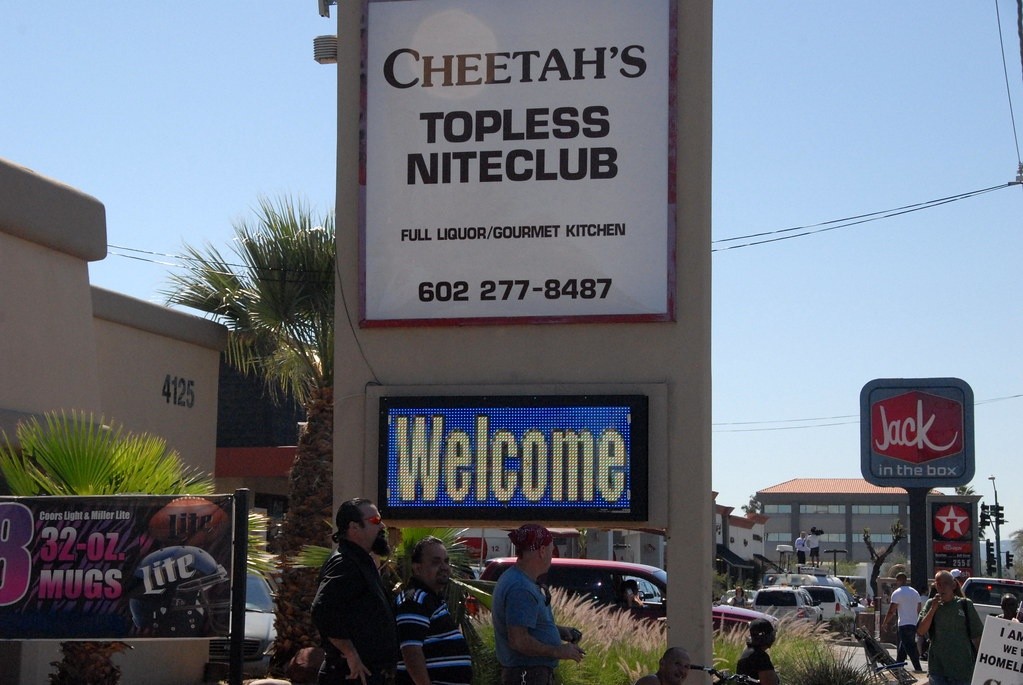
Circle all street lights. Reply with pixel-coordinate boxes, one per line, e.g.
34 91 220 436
988 477 1002 578
824 548 848 578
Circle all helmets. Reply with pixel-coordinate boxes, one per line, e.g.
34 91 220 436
130 546 231 637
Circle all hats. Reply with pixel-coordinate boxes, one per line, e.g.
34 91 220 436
749 618 773 639
951 569 961 578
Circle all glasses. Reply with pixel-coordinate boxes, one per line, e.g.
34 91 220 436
362 516 381 526
537 581 551 607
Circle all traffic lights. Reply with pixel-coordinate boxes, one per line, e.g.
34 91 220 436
1006 550 1014 569
986 539 996 576
996 504 1004 524
981 502 990 525
990 505 996 516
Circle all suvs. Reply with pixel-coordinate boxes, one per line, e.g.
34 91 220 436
751 586 822 627
961 577 1023 625
800 585 858 623
480 556 778 639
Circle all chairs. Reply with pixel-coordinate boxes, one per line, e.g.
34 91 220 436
974 589 990 604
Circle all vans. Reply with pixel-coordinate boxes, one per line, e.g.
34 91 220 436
764 572 866 613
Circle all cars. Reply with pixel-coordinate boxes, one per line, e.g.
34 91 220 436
721 589 757 609
208 568 279 675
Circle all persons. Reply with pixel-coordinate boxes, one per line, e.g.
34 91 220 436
489 523 587 685
633 647 690 685
392 535 472 685
730 586 747 608
620 579 644 607
995 593 1023 623
915 570 984 685
311 498 397 685
916 569 966 659
857 594 872 607
806 526 819 568
736 618 780 685
881 572 923 672
795 531 807 574
